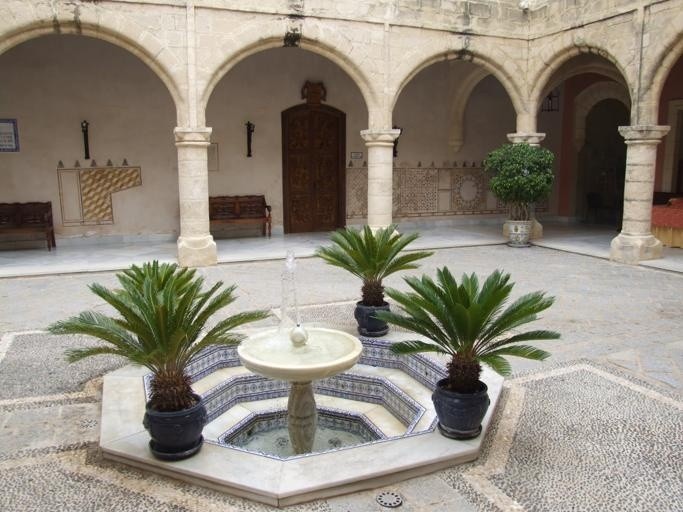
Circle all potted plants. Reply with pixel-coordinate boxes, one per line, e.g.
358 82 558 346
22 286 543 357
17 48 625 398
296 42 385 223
370 266 562 442
45 261 273 462
484 142 555 248
314 225 435 336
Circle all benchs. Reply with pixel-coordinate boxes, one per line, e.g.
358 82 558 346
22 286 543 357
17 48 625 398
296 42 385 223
0 202 56 252
208 195 272 238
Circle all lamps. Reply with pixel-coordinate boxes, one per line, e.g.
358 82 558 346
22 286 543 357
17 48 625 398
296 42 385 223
81 120 90 159
393 126 402 158
245 122 254 157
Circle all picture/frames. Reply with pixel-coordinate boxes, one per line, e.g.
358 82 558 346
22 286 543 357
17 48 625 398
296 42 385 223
206 142 219 171
